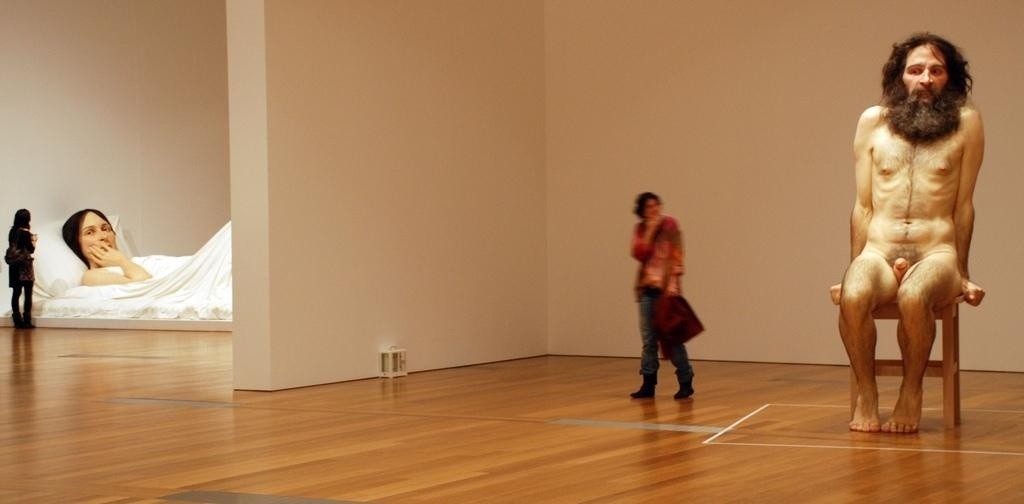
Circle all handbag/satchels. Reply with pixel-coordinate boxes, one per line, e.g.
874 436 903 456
655 266 705 357
5 248 34 271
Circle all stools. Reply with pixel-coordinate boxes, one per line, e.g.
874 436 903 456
849 288 967 428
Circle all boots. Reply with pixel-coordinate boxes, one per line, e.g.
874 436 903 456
12 311 36 329
629 371 657 399
673 370 696 400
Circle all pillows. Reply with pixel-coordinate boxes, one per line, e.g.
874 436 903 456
8 216 133 293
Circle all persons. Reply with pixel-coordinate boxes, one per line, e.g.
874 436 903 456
62 210 152 286
838 33 985 434
8 209 35 329
630 192 694 399
